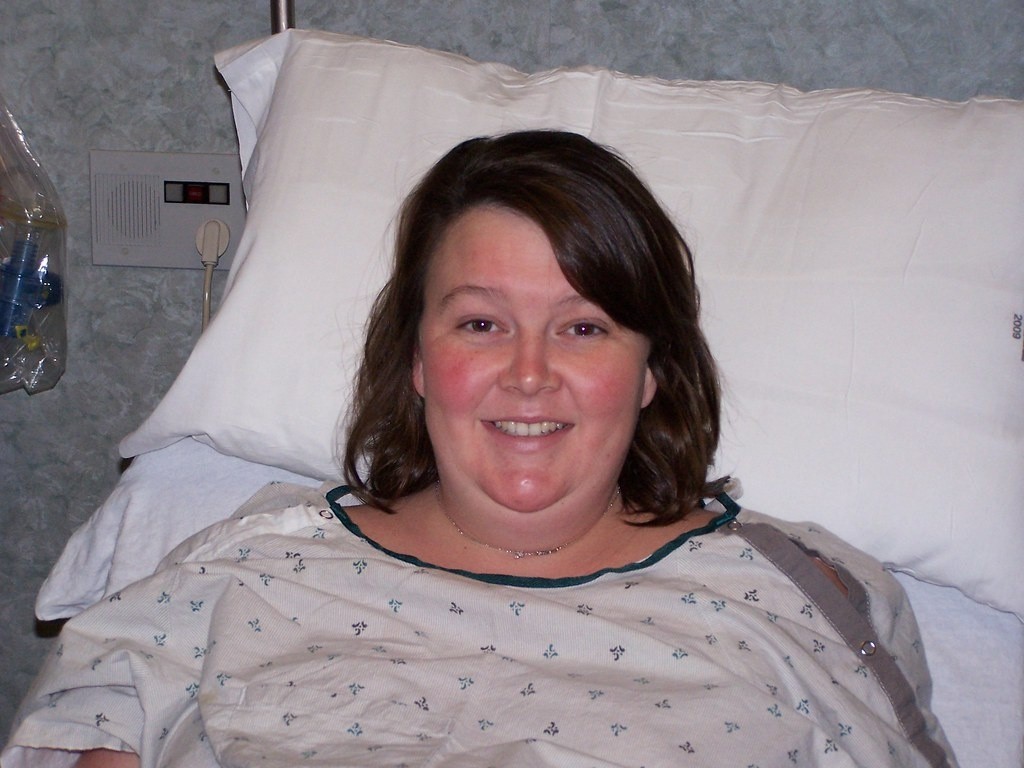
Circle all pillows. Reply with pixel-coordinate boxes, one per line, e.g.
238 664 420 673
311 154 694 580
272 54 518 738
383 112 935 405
30 433 1024 768
118 26 1024 582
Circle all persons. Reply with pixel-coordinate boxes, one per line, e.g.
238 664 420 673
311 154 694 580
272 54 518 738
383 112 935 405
1 131 958 768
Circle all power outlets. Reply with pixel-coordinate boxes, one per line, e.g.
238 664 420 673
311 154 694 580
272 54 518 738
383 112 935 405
90 149 246 271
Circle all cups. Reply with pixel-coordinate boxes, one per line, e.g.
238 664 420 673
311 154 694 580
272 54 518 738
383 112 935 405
0 204 59 338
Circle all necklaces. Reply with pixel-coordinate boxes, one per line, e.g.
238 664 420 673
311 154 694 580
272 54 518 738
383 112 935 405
436 481 620 559
